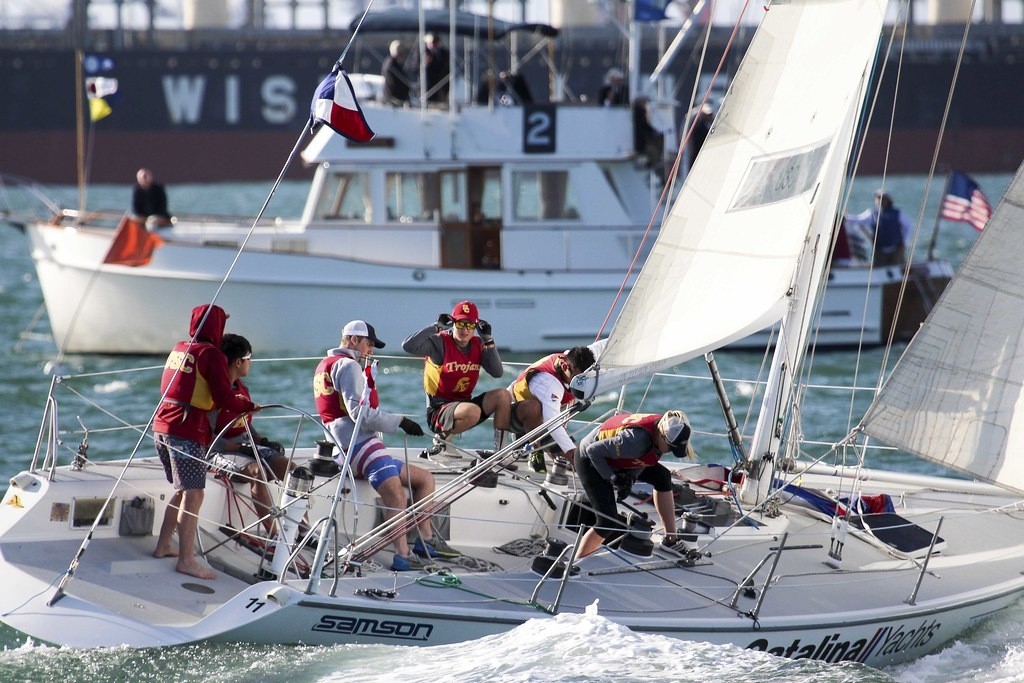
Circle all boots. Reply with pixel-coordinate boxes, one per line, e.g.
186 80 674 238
432 429 464 458
494 428 518 470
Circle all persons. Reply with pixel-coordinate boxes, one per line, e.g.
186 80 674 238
152 305 264 579
633 97 673 186
130 168 171 232
857 189 912 266
412 31 449 102
575 411 693 561
495 72 532 106
313 321 460 571
833 215 857 265
384 40 412 107
504 346 594 473
203 333 321 548
402 301 518 470
599 69 628 107
693 104 713 157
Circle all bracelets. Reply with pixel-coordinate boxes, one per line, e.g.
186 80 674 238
485 341 494 346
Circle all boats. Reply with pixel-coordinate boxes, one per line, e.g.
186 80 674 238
7 0 969 357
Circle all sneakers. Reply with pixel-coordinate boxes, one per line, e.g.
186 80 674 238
391 549 437 571
413 534 461 557
659 535 689 558
528 449 546 473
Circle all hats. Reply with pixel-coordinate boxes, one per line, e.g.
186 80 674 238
452 300 479 324
342 320 385 349
661 415 690 457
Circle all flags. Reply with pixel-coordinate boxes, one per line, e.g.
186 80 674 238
84 55 113 76
89 97 112 121
940 167 990 231
86 75 117 98
103 218 165 268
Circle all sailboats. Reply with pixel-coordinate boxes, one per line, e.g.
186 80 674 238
0 0 1024 669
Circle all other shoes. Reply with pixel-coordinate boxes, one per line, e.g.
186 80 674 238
297 534 318 548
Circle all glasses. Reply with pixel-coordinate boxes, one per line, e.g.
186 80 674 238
239 353 252 361
453 321 478 329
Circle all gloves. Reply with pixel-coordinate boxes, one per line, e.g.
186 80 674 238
614 474 636 500
399 418 424 436
